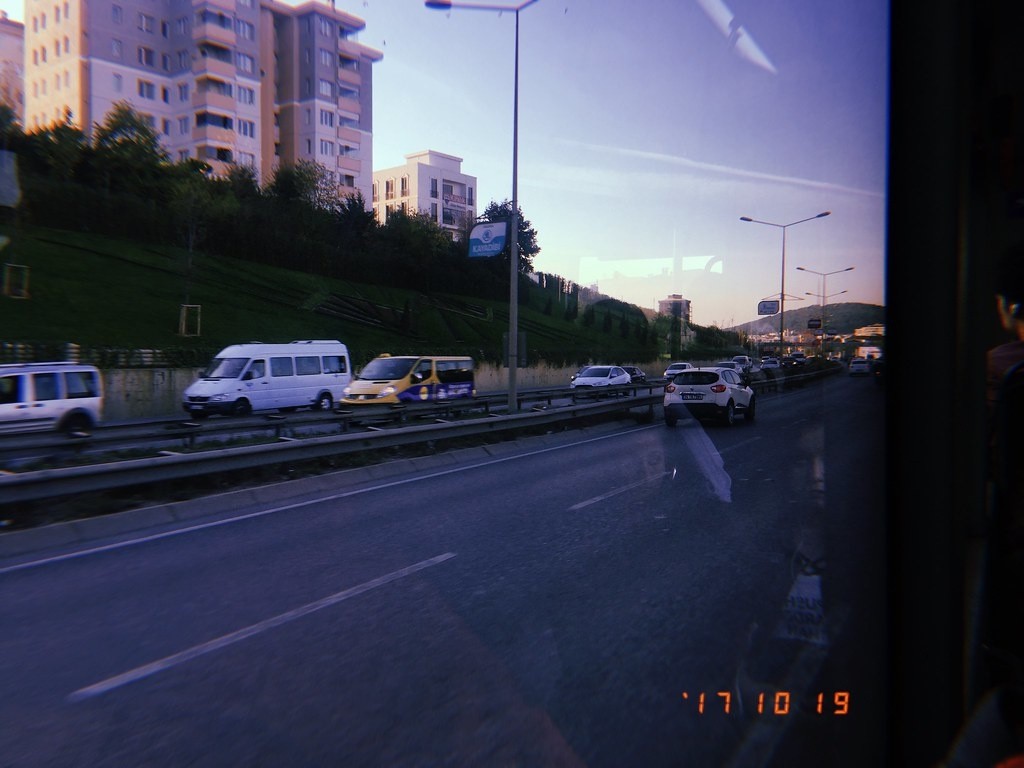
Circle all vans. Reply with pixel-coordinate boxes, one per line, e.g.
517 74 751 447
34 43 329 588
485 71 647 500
337 352 477 407
0 363 104 440
183 339 352 419
788 352 804 362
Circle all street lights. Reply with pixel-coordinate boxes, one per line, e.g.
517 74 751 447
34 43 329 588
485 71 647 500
797 266 855 351
424 1 519 414
806 291 847 353
740 211 831 354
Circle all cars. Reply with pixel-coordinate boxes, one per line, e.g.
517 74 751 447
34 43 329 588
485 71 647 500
622 366 647 386
663 366 756 427
760 359 780 370
714 362 743 374
731 356 753 369
663 363 695 383
570 366 591 381
781 357 799 369
831 356 871 374
569 364 632 399
761 356 770 363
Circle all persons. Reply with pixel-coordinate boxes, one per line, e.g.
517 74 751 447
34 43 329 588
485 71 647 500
976 245 1023 748
